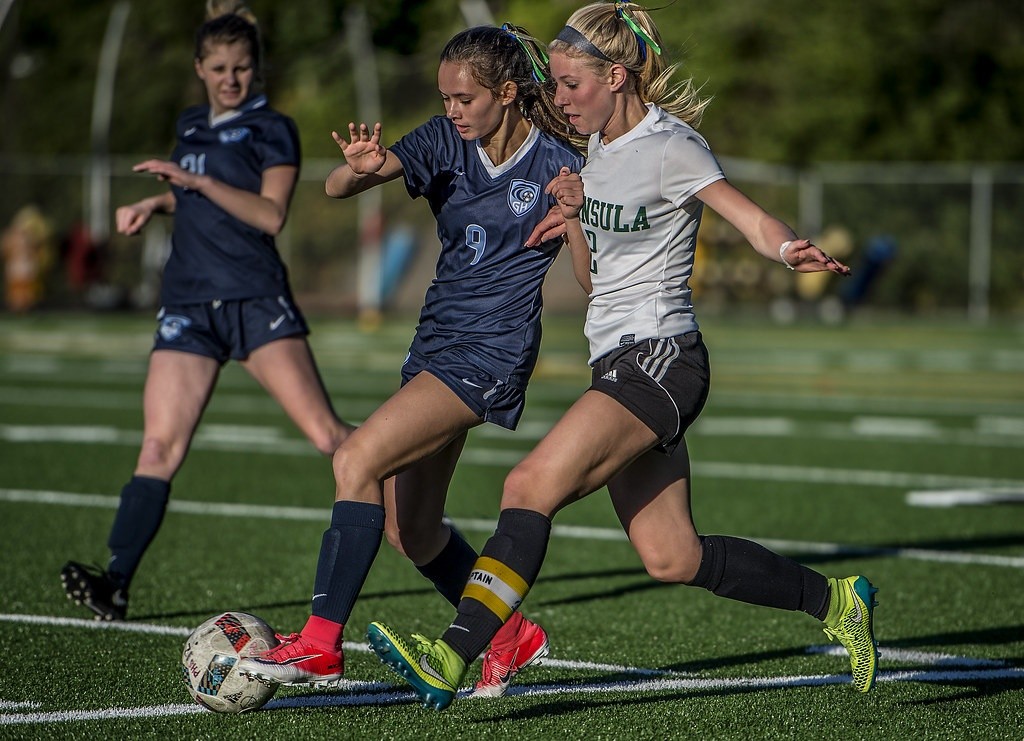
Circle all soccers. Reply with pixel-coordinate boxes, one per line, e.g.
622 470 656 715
183 613 282 713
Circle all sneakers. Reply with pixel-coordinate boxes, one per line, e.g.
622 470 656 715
237 632 344 688
365 621 469 711
464 617 550 699
822 574 882 692
61 560 130 622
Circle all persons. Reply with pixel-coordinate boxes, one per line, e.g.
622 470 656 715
60 0 406 622
364 1 883 713
238 22 587 697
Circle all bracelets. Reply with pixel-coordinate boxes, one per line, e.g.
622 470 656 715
779 240 795 272
